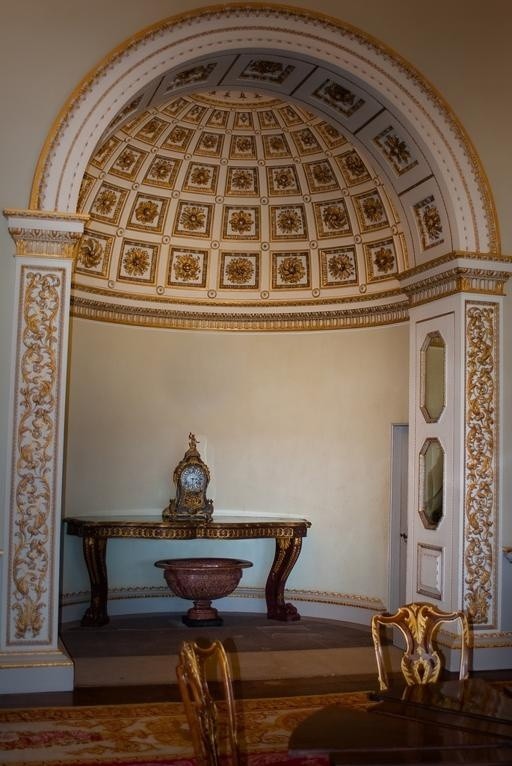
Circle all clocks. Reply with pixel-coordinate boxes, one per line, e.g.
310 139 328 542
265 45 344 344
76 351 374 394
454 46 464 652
162 432 213 522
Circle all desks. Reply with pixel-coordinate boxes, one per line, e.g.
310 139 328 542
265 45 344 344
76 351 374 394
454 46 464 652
63 515 311 626
288 677 512 766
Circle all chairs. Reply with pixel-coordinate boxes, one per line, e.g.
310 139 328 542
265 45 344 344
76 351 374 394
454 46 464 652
176 639 239 765
372 602 474 689
